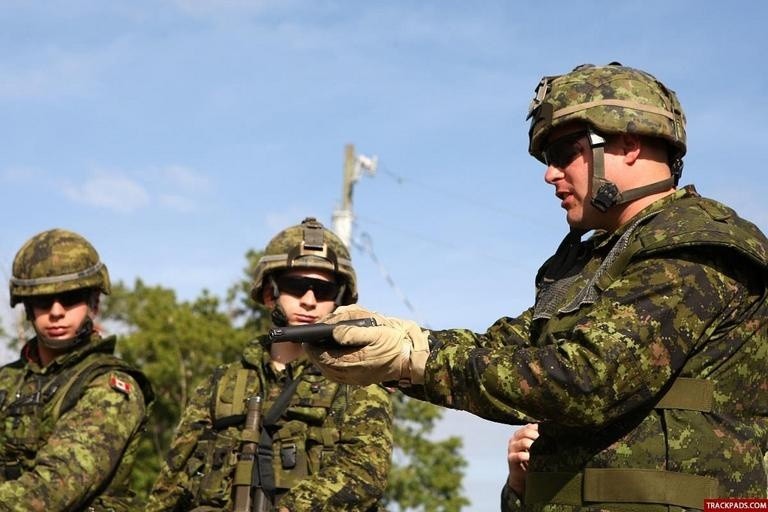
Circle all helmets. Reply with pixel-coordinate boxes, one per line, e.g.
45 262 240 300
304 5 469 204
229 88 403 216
539 129 592 168
7 229 112 307
525 67 689 157
248 220 360 305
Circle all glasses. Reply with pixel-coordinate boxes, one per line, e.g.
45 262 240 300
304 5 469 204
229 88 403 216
273 273 340 302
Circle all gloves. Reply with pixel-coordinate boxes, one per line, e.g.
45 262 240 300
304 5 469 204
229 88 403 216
300 306 433 389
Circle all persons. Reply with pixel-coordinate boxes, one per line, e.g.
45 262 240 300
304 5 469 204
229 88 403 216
145 215 395 511
1 226 158 512
302 60 767 512
498 421 540 512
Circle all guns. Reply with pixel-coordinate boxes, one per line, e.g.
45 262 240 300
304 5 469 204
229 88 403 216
266 316 379 359
